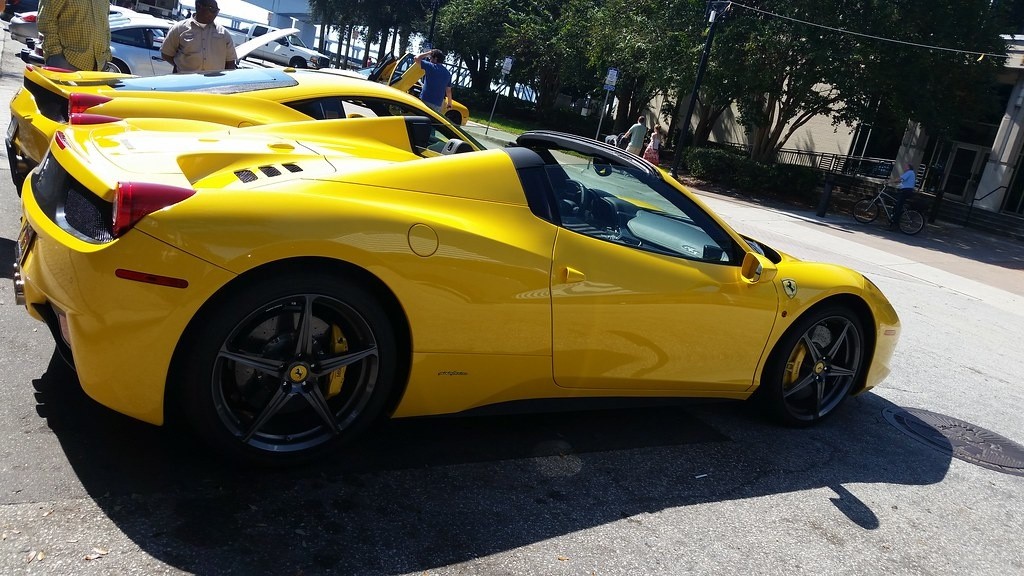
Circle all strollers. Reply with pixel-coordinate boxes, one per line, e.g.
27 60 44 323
604 132 629 149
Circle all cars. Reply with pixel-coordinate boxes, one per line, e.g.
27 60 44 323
5 4 300 74
301 51 471 134
2 63 490 199
10 110 904 490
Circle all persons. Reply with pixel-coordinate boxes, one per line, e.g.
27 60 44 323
414 49 452 136
36 0 112 72
643 124 661 167
622 116 646 155
159 0 238 73
885 162 916 231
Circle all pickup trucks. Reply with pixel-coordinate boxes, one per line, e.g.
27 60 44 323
222 23 332 69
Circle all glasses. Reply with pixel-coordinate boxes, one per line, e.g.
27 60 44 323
202 5 221 14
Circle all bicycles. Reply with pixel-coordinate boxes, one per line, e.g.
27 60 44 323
851 182 925 235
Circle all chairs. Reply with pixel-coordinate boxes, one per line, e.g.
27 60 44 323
440 138 553 221
145 30 154 49
